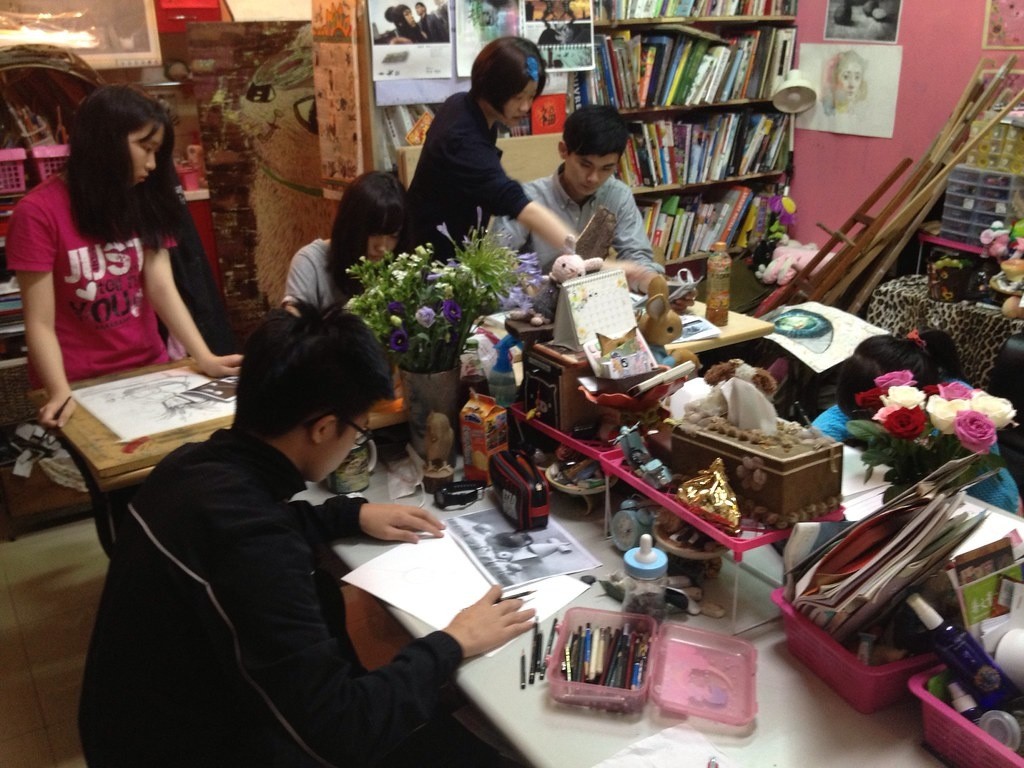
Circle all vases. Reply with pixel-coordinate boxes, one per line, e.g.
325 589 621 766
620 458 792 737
394 367 457 463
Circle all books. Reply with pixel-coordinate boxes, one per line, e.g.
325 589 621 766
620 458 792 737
611 112 790 188
782 449 1024 649
593 0 798 21
384 104 446 173
574 24 797 111
638 172 789 260
495 111 532 137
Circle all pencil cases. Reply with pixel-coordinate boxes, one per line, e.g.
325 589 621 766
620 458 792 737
486 446 550 532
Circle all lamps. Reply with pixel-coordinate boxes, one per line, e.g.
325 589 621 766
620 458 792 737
770 69 818 199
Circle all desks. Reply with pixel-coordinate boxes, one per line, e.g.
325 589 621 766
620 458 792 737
291 446 953 768
659 300 777 382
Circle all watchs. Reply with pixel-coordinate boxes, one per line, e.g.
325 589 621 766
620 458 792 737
435 480 486 509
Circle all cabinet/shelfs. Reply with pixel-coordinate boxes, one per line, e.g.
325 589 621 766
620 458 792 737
567 14 797 283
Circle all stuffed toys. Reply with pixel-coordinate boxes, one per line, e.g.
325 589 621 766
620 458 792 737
636 276 703 369
661 359 778 421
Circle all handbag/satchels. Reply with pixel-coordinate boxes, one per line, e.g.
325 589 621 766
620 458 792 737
489 448 551 530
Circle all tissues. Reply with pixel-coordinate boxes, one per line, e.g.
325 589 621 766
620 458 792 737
670 375 843 519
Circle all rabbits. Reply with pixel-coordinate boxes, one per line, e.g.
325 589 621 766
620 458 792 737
636 275 701 367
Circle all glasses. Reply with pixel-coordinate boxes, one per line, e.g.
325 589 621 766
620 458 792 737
304 410 374 445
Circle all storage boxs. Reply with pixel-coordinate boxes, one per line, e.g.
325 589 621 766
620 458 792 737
510 398 1024 768
911 232 1016 310
153 0 234 83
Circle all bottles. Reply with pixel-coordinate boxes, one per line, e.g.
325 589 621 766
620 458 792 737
704 242 731 325
622 534 668 636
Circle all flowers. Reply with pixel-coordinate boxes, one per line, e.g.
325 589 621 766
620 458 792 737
347 209 535 377
843 366 1015 489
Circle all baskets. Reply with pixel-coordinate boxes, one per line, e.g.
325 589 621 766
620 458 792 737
1 148 28 195
907 646 1024 768
32 144 72 183
0 336 43 423
769 585 953 715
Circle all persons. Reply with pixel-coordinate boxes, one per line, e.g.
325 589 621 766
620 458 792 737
486 104 665 294
538 22 590 42
282 170 413 317
394 37 579 270
804 327 1024 517
415 2 449 41
6 83 244 560
78 296 536 768
384 4 427 44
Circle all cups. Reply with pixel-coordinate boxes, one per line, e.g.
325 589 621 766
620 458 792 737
327 434 376 493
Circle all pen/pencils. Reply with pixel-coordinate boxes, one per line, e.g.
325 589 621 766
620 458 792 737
518 646 528 691
528 614 558 684
492 590 536 604
561 622 650 689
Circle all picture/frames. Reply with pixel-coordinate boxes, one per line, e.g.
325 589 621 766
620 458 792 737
26 354 242 478
